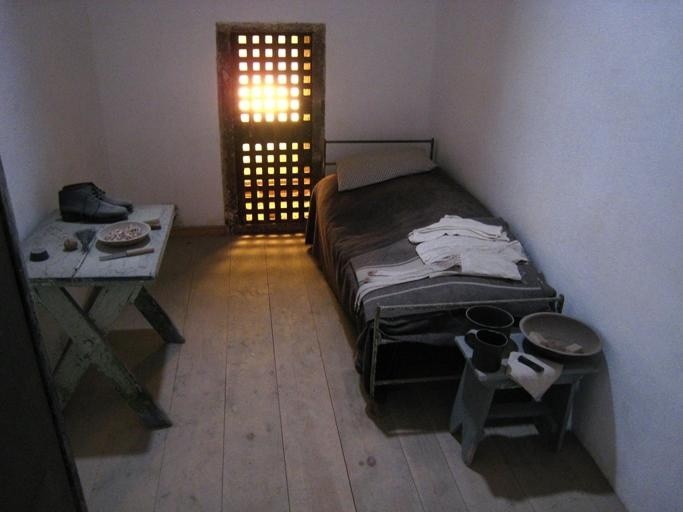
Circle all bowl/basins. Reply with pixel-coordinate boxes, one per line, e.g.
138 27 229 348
464 304 514 330
519 311 604 360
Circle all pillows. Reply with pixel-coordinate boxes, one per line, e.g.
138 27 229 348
334 142 439 193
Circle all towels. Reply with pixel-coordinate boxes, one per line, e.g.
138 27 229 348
503 348 564 405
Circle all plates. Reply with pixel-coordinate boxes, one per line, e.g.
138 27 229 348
96 219 151 246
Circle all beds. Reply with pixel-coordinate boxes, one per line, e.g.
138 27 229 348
318 134 567 419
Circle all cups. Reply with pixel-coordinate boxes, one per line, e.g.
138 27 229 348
464 330 508 374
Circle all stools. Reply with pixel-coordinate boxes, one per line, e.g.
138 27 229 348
445 328 600 469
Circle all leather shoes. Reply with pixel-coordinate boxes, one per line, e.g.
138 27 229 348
59 189 128 223
63 182 134 213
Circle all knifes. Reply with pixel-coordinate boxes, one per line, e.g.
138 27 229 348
99 247 154 262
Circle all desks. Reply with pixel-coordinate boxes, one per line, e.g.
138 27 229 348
19 202 187 427
